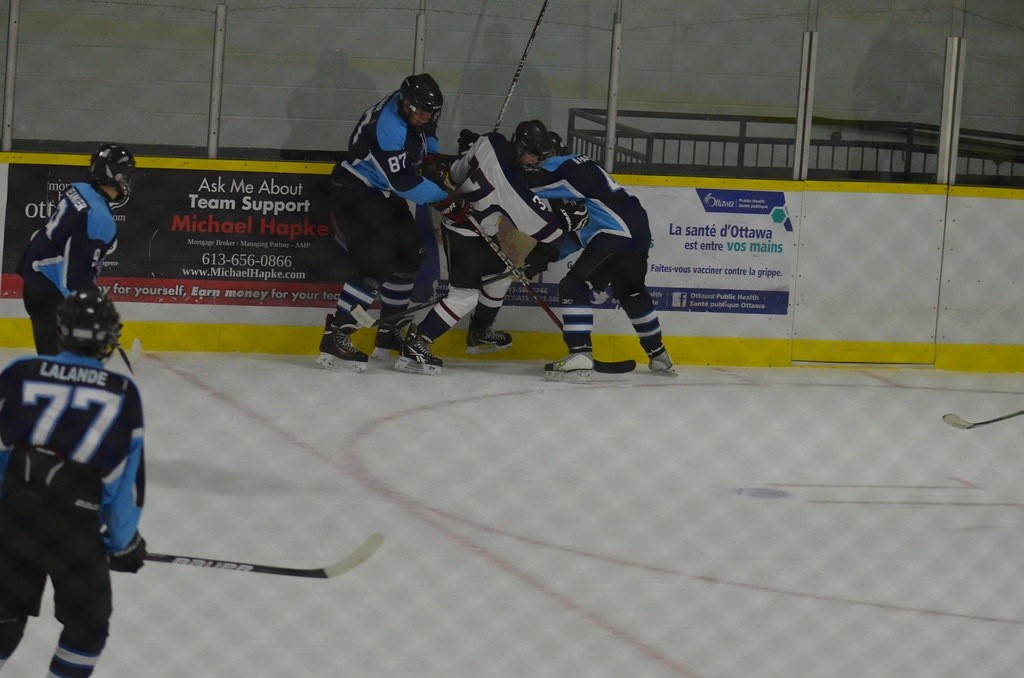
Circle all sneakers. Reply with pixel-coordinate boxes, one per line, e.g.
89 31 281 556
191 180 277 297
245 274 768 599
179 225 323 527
467 329 514 348
648 347 679 377
543 347 594 372
373 324 406 355
399 334 442 371
321 319 368 362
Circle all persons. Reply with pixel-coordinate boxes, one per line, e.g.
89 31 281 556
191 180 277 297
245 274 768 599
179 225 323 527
315 73 473 373
395 119 590 376
0 288 147 678
523 133 679 385
14 143 137 357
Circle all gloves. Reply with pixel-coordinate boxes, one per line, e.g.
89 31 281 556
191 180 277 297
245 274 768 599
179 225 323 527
559 202 588 235
524 247 550 280
423 157 447 183
433 190 476 222
106 533 150 575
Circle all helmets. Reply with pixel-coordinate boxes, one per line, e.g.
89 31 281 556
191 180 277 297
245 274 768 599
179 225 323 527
398 73 443 134
514 120 552 174
55 290 122 362
91 143 137 187
548 132 569 157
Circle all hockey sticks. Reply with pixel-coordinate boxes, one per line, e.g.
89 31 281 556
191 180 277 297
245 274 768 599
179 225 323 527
118 338 143 375
493 0 548 132
467 213 637 374
143 531 384 580
943 408 1024 430
349 261 534 330
